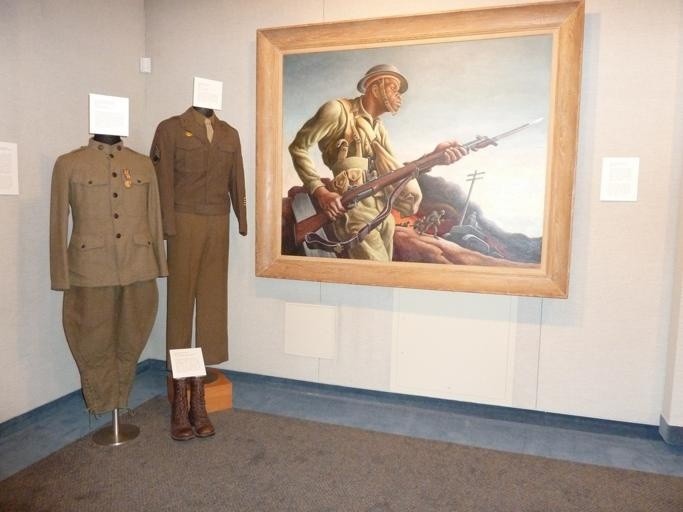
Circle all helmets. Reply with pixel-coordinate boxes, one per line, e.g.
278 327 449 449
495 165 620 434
357 65 408 94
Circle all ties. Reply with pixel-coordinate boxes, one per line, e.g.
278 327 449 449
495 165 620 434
205 118 213 142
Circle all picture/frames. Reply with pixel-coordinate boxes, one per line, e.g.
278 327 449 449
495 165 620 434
251 2 585 302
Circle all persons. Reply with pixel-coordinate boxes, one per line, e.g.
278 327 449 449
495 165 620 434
150 107 247 371
50 135 168 420
288 64 467 262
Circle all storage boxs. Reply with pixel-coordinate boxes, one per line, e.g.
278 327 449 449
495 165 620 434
164 369 234 418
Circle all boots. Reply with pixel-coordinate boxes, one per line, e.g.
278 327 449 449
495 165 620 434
172 378 215 441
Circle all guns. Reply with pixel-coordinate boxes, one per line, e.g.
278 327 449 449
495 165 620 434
293 117 546 245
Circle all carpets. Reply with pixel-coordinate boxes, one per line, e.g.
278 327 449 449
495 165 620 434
0 392 683 512
